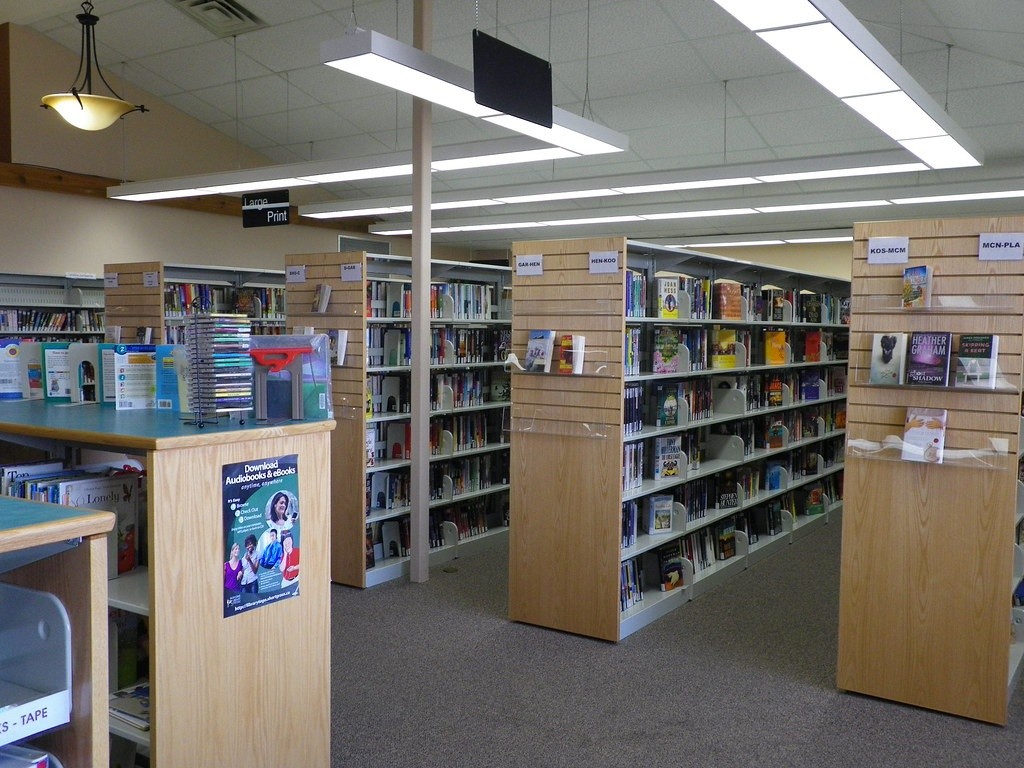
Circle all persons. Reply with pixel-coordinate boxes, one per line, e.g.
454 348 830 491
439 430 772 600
224 492 298 607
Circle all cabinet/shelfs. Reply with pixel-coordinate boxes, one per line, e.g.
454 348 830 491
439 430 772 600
835 215 1024 729
0 271 104 345
0 495 117 768
284 251 513 590
0 396 337 768
506 235 851 644
103 261 287 345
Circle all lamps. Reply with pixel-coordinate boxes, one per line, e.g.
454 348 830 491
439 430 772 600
107 0 1024 250
38 0 151 132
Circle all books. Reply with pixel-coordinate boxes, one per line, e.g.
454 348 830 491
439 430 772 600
524 329 557 373
869 331 908 386
558 334 586 375
1 306 105 343
954 333 999 389
907 331 954 387
162 275 285 350
1 342 197 410
2 461 153 768
363 265 512 570
1010 387 1024 605
902 266 935 311
901 406 948 463
620 253 852 619
